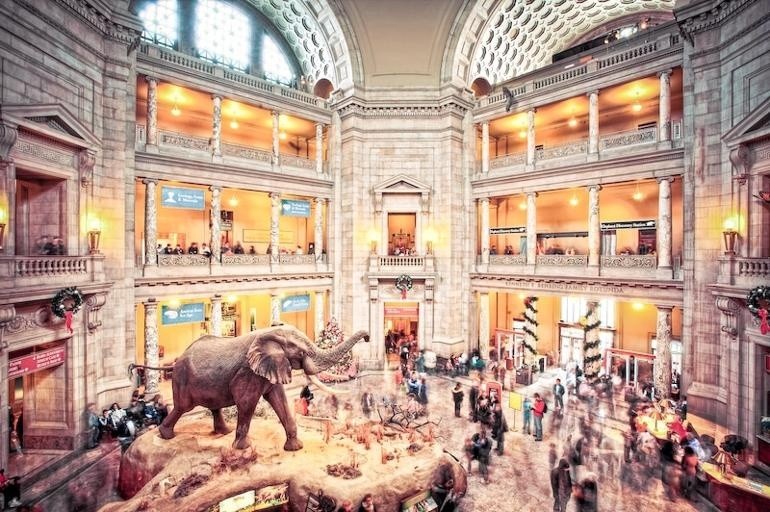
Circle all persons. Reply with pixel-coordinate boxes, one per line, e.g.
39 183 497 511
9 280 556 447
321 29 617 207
0 468 44 511
503 242 577 256
384 328 711 510
156 244 162 263
174 244 183 254
30 235 65 256
296 245 303 254
299 381 312 416
199 243 211 255
220 239 256 255
164 243 172 253
308 244 315 254
490 245 497 254
638 240 646 255
360 386 377 420
188 242 197 254
85 388 169 454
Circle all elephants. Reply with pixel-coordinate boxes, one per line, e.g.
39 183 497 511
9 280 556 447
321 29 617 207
127 322 370 452
